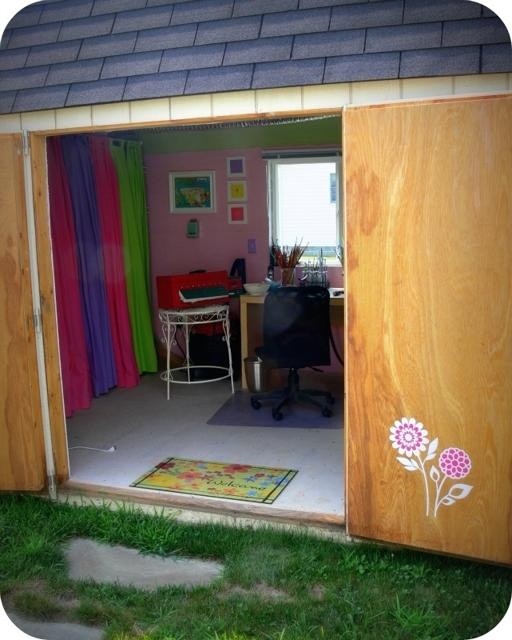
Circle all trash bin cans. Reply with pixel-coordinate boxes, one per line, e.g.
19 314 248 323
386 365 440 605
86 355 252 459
245 357 267 393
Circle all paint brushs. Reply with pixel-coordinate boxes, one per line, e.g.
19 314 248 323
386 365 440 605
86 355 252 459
271 237 309 285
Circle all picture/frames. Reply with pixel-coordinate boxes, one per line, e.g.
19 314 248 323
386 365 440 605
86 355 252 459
168 170 216 214
225 156 248 225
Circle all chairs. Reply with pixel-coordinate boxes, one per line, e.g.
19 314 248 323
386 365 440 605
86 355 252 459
251 286 336 420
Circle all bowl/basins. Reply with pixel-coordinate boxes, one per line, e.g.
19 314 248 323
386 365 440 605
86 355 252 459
243 283 269 295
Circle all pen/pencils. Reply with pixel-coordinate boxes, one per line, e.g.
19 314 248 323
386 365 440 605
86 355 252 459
304 248 328 286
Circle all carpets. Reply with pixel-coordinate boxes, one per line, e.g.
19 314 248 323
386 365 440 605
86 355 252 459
130 457 299 504
207 390 344 430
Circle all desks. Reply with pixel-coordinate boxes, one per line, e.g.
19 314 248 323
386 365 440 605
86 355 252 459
160 302 235 400
239 287 343 389
